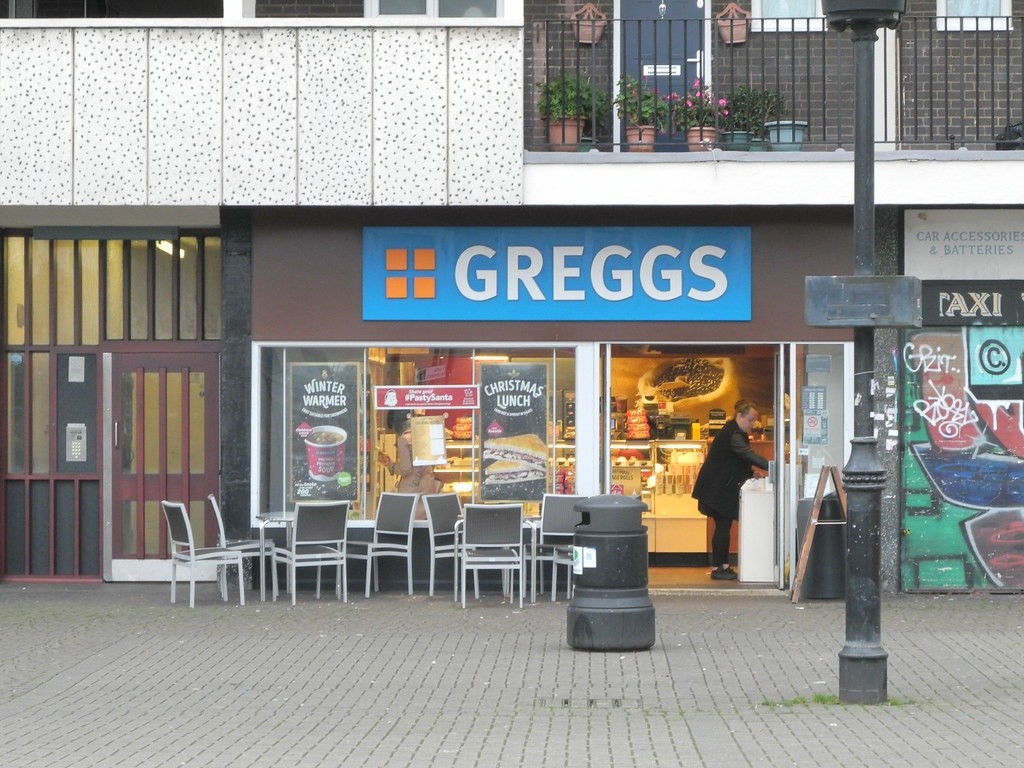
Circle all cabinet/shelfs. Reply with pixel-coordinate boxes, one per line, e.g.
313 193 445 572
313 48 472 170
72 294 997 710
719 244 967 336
738 489 773 583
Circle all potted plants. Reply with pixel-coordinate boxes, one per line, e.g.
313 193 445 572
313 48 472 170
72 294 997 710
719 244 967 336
718 85 808 152
536 70 608 151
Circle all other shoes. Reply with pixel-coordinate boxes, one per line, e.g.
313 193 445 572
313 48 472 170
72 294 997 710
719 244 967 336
711 565 737 580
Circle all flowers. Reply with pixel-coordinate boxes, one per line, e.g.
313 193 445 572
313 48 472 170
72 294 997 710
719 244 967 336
667 76 729 134
610 72 669 136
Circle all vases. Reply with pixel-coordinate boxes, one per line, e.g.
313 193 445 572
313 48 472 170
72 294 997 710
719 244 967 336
625 126 659 151
686 126 715 151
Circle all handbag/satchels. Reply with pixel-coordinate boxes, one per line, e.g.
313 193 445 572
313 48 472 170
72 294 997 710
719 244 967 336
434 478 444 494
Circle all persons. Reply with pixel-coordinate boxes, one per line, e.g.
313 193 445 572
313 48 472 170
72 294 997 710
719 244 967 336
383 411 438 520
690 399 769 580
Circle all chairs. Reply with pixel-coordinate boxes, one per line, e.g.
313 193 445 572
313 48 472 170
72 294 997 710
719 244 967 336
271 499 349 606
421 492 510 598
160 498 245 608
208 493 280 601
522 493 591 602
334 492 420 597
460 503 525 610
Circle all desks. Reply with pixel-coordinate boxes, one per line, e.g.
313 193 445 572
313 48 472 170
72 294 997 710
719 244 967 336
453 515 541 603
255 511 341 600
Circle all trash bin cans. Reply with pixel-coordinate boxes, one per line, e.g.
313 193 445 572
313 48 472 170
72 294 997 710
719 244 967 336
796 496 847 600
566 495 656 651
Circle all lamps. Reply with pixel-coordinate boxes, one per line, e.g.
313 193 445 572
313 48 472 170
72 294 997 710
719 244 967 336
659 0 667 19
